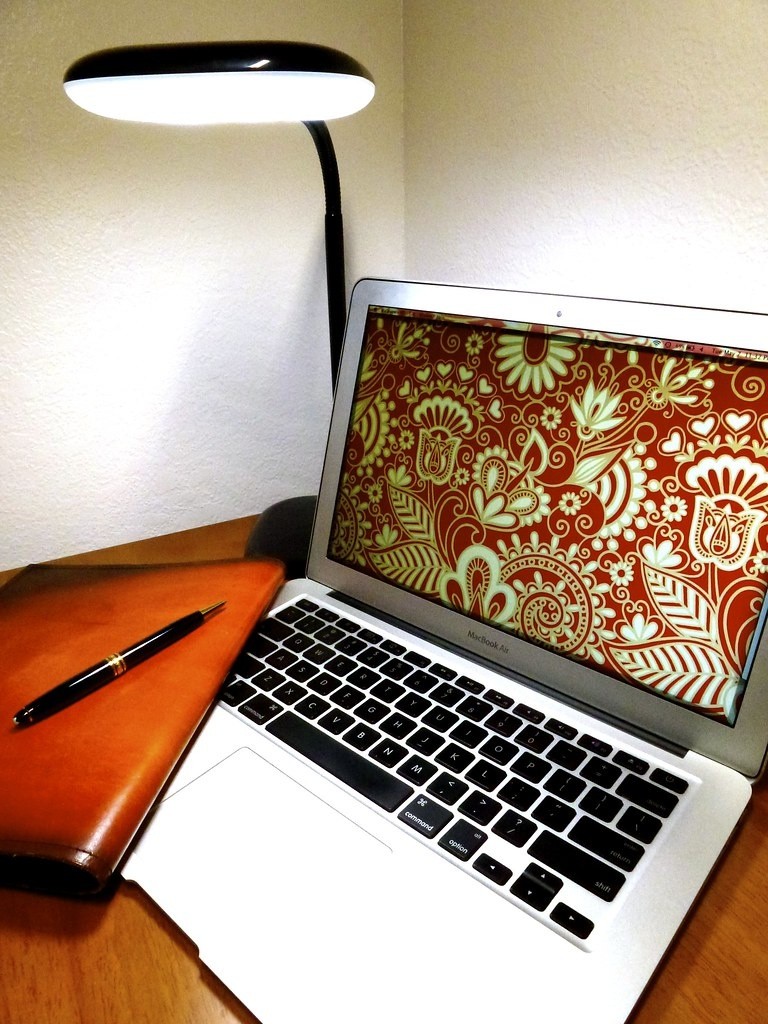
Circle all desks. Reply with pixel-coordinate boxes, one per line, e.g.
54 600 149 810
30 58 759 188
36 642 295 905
0 510 768 1024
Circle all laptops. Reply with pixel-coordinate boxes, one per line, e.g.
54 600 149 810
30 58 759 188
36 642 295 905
113 274 768 1024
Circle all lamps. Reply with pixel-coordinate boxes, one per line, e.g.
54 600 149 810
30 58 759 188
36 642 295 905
63 34 382 580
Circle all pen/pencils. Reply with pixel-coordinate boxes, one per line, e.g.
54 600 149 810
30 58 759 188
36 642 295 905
14 600 227 726
